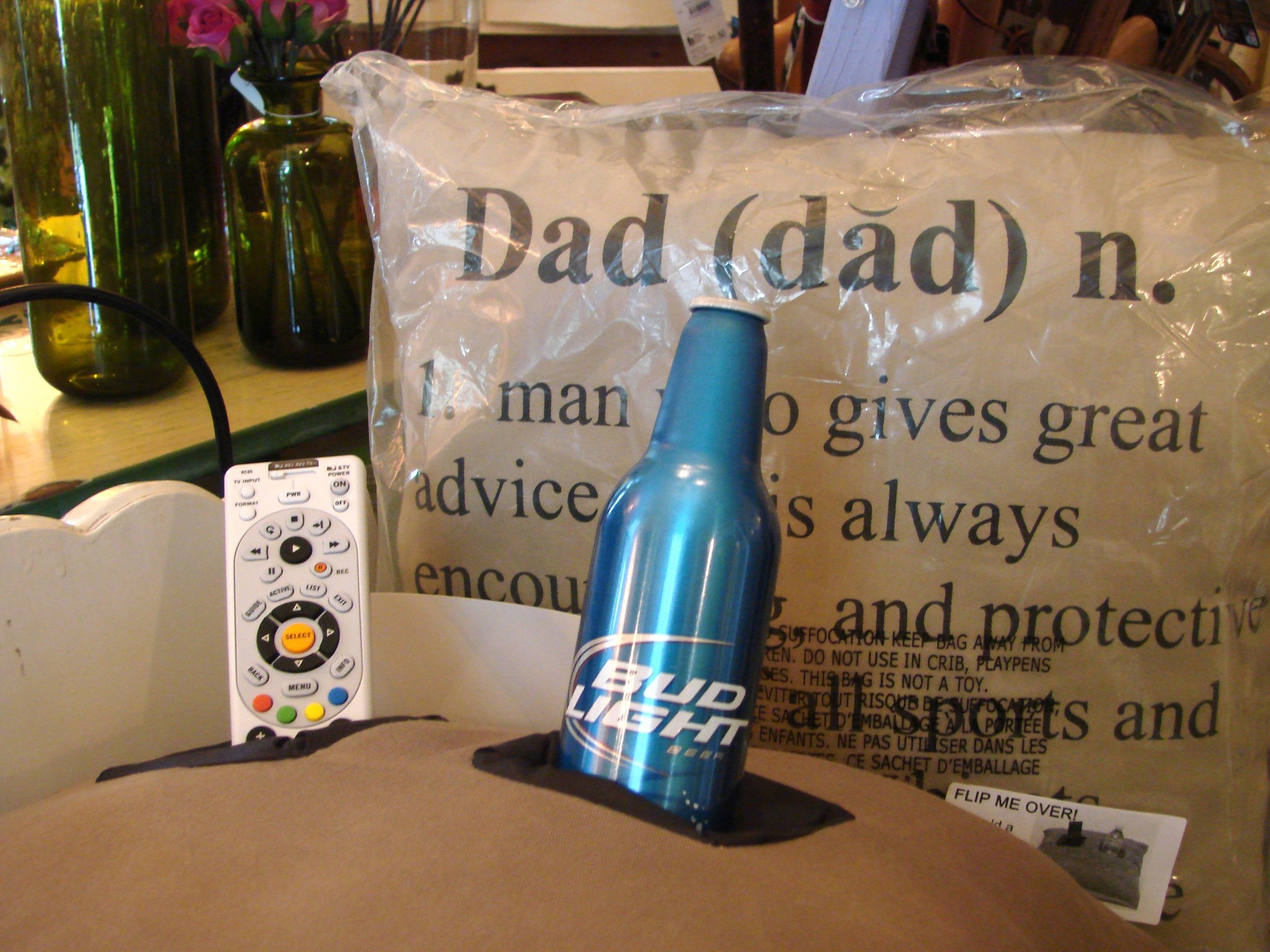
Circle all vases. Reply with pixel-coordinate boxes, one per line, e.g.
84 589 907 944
0 0 374 402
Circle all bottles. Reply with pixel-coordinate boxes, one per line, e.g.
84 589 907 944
0 0 197 403
559 295 784 834
170 47 230 333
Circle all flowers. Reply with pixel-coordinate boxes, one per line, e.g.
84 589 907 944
155 0 348 90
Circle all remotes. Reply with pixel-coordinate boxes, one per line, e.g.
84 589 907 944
221 454 371 747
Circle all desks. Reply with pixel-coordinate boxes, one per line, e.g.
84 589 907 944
0 213 403 523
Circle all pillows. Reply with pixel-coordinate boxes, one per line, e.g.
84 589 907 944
322 43 1270 952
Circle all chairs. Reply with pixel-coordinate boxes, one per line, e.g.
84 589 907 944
0 481 582 803
